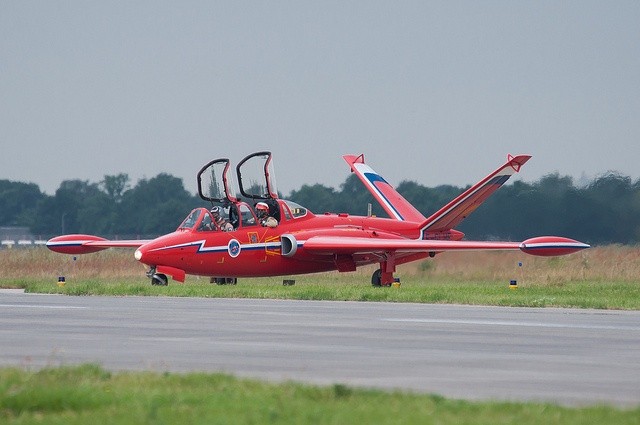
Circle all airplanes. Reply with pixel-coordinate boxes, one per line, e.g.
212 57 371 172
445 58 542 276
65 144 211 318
46 152 590 287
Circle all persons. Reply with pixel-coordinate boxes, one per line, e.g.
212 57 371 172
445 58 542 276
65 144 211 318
247 202 279 228
202 206 235 233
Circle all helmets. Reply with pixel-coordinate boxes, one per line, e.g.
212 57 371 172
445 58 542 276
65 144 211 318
210 205 225 223
256 202 269 219
